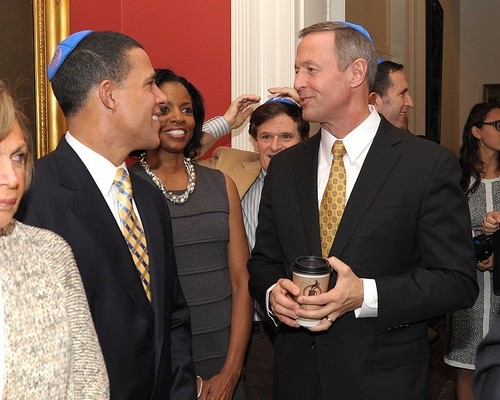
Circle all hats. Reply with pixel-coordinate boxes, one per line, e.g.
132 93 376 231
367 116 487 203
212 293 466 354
267 97 296 104
48 29 93 82
377 58 384 65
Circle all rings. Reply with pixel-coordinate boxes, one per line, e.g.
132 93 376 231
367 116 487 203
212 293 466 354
325 317 333 323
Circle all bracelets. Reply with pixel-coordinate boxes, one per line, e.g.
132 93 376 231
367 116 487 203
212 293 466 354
196 376 203 398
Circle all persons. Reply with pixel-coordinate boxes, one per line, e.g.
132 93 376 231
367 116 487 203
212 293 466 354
127 68 254 400
368 60 414 128
14 30 196 400
245 21 480 400
189 87 310 400
444 102 500 400
0 80 110 400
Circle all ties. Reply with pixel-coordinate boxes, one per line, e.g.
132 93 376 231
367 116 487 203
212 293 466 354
112 168 152 303
340 21 373 43
319 140 347 259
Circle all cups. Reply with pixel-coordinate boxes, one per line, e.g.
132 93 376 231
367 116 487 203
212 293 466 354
290 256 334 327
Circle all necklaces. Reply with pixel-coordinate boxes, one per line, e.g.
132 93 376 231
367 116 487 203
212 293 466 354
141 157 196 204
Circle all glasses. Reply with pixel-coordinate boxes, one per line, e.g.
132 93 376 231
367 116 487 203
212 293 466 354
478 121 500 132
